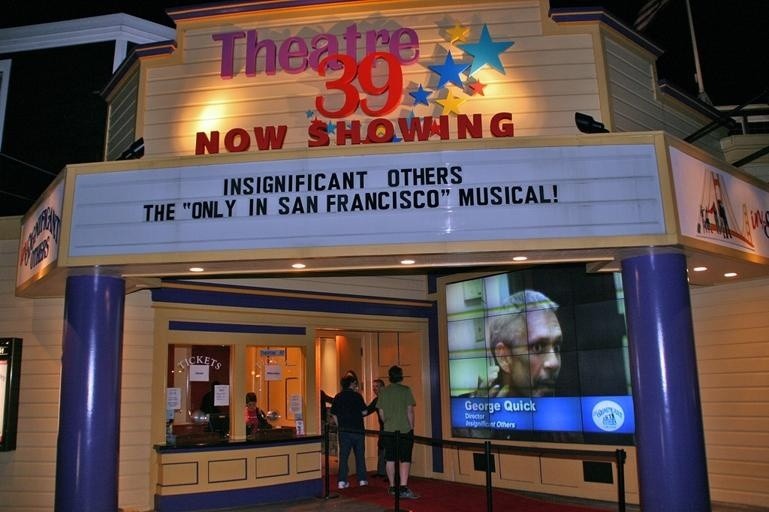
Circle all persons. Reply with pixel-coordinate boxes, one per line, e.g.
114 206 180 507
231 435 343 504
468 289 563 399
321 389 336 422
374 364 421 499
331 375 370 490
242 392 265 426
361 378 389 482
342 369 359 392
197 379 222 433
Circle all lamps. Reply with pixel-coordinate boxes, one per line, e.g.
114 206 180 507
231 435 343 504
575 112 609 133
116 137 144 160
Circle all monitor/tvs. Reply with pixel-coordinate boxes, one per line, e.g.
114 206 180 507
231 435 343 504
207 414 229 441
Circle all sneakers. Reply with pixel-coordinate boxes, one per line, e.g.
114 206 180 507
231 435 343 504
400 489 420 499
387 486 396 496
338 481 349 489
360 480 368 485
